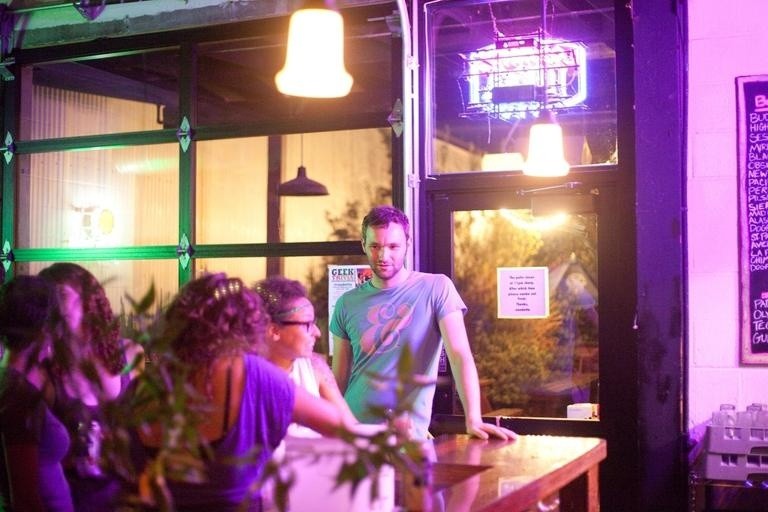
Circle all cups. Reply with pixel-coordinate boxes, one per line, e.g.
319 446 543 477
710 402 768 439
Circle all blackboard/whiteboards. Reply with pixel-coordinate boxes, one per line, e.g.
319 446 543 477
735 74 768 366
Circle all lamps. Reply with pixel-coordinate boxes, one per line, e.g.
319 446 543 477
521 1 570 177
274 7 355 197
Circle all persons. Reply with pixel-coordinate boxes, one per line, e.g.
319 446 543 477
329 205 519 456
106 273 341 512
36 262 146 478
0 273 78 512
250 276 359 442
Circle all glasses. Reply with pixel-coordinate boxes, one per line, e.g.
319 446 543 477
281 318 316 331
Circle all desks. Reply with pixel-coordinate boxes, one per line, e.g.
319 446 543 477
435 434 608 511
